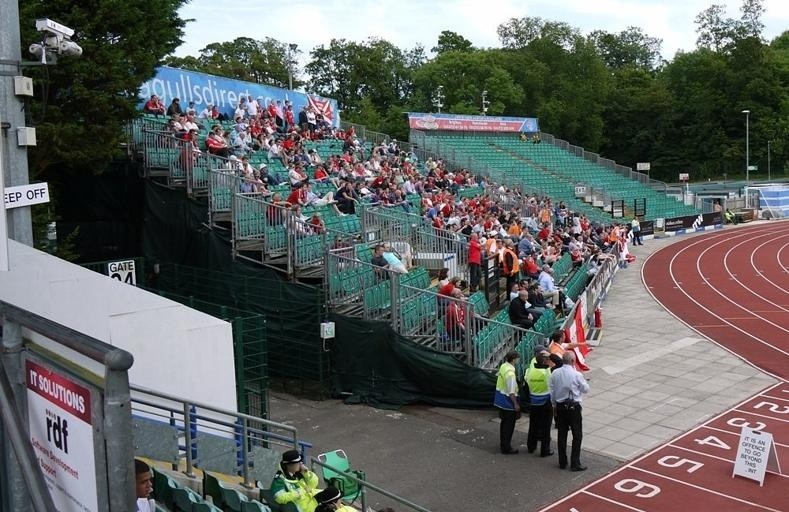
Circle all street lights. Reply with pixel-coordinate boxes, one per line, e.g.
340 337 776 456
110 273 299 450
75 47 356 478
482 90 490 115
431 86 446 113
742 108 750 186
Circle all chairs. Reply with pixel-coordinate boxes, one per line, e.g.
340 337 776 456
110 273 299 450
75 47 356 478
193 169 337 190
203 470 269 512
149 114 173 129
173 163 327 176
152 464 219 512
149 148 372 165
148 141 374 150
199 126 232 130
241 207 330 237
354 185 484 212
318 450 363 506
337 222 442 281
414 135 704 235
199 120 233 125
448 253 575 345
271 214 421 258
198 133 231 142
368 240 465 317
480 267 588 373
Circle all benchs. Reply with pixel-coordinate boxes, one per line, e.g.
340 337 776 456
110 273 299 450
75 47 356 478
297 210 419 248
215 174 333 197
402 256 464 340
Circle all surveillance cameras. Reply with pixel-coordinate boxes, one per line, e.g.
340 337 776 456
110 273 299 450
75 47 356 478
29 17 82 64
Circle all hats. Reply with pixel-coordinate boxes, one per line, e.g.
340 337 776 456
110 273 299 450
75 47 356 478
209 130 219 135
282 450 304 464
319 486 344 504
534 345 551 351
309 149 317 153
259 163 268 171
230 155 238 161
303 105 309 110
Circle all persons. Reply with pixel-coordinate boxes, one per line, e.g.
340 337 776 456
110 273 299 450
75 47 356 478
206 132 228 163
311 213 324 234
632 217 643 245
550 351 589 470
223 132 237 152
207 125 226 133
326 128 362 182
381 244 407 277
520 280 530 289
157 113 206 169
331 236 362 270
522 350 563 457
267 99 277 118
247 96 258 118
425 157 523 239
215 130 226 144
234 104 245 118
307 184 348 216
438 276 462 305
335 183 359 215
289 167 309 187
467 234 488 292
361 140 427 215
286 184 310 208
494 352 522 453
284 101 289 117
276 101 283 125
315 164 341 189
550 330 587 428
503 240 519 299
298 107 308 124
450 289 488 328
283 206 311 237
314 487 343 512
267 193 292 223
286 106 293 125
259 164 288 186
270 450 319 512
227 118 333 164
143 96 164 118
446 295 478 343
200 106 212 119
520 193 627 280
439 269 449 288
725 208 737 225
530 346 553 367
509 291 536 327
307 107 316 125
135 459 154 512
236 99 245 105
509 282 544 317
167 97 184 115
186 103 196 116
539 269 575 309
527 284 555 309
224 156 271 198
213 106 228 121
714 202 722 211
371 246 400 281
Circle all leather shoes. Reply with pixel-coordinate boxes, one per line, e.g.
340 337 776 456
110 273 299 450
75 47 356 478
542 450 555 457
633 244 637 246
572 463 588 471
528 445 537 453
560 460 567 469
505 447 520 454
638 242 643 245
